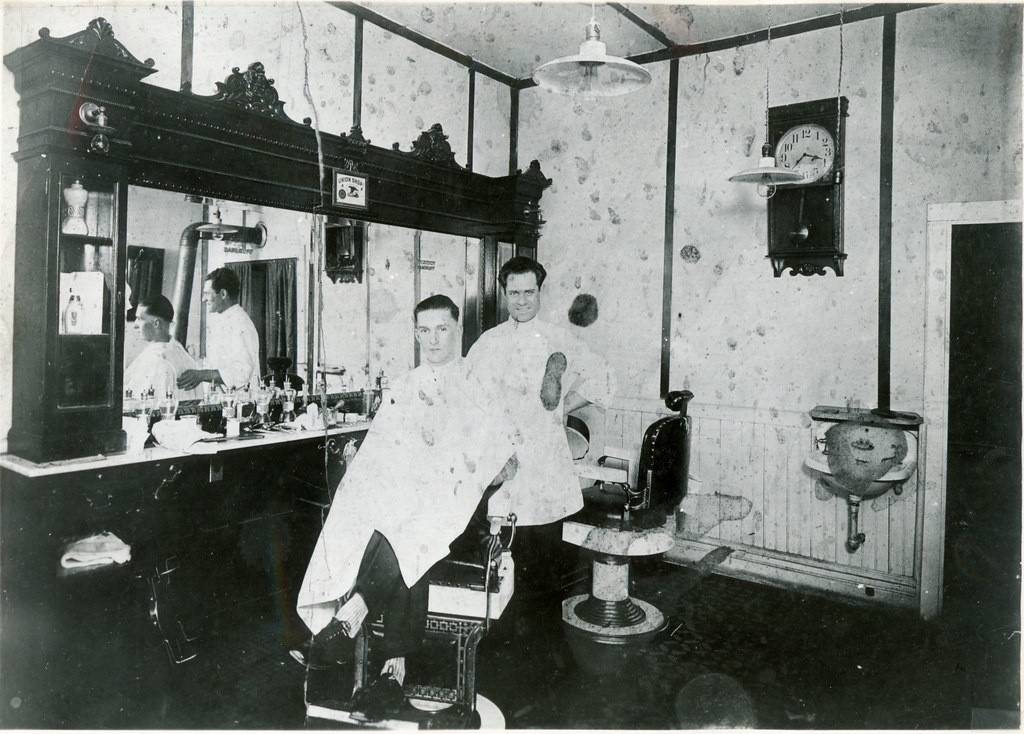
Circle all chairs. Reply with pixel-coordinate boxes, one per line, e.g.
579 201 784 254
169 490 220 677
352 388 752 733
561 389 694 638
304 436 515 729
261 357 305 392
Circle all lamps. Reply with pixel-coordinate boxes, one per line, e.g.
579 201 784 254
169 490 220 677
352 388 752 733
195 199 238 241
79 102 117 154
532 2 652 98
729 4 804 198
524 205 548 237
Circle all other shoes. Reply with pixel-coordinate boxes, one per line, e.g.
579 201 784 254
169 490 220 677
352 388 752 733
514 610 540 636
549 635 569 672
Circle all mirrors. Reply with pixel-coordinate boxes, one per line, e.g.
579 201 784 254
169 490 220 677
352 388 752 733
113 178 485 453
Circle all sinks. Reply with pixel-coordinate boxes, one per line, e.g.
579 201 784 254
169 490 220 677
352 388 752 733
805 448 915 500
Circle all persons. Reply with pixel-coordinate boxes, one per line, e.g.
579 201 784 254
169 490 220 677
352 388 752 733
465 257 618 684
123 295 204 412
287 294 521 723
176 268 260 392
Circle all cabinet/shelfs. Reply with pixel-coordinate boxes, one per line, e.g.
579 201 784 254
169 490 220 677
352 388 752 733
9 155 126 463
0 430 370 730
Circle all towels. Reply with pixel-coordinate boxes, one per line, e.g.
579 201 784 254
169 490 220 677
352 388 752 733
60 532 132 569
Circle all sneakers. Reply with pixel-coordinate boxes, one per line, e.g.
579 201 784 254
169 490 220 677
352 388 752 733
349 665 404 721
288 616 356 669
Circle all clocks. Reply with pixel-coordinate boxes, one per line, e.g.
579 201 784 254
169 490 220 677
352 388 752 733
764 94 849 279
